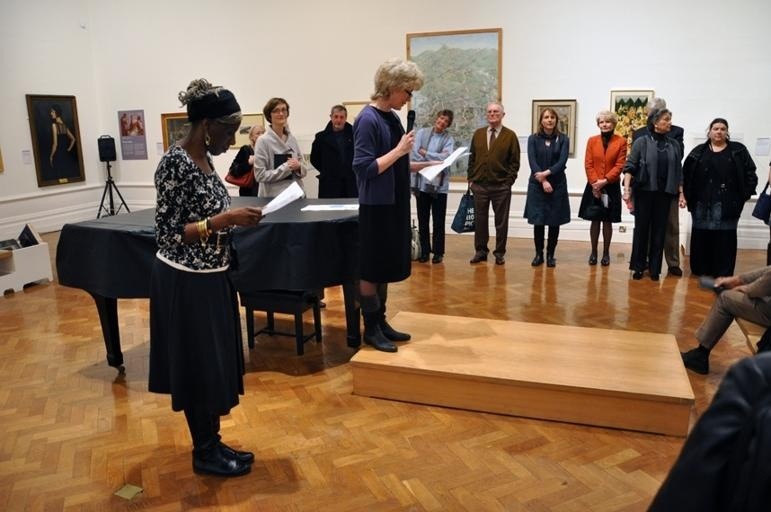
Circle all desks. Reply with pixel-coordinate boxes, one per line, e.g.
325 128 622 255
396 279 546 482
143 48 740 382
56 195 359 367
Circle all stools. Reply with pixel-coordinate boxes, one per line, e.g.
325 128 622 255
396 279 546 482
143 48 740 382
239 287 325 356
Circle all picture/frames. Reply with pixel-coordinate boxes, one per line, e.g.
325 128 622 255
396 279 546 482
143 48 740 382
406 27 502 182
531 100 577 159
610 89 655 114
25 94 87 187
161 112 189 153
227 113 264 150
343 102 372 125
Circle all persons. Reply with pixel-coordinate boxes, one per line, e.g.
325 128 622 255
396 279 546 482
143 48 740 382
352 59 444 353
578 110 629 267
121 113 145 137
409 109 457 266
49 105 77 178
148 77 267 477
522 106 570 269
310 105 359 201
621 95 687 282
646 349 771 511
466 102 520 265
227 97 328 309
682 117 759 294
679 265 771 377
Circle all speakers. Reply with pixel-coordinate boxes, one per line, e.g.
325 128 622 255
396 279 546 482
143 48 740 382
98 135 116 161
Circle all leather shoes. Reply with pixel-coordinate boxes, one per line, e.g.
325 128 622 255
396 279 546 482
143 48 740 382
192 444 254 477
679 351 709 375
418 253 682 281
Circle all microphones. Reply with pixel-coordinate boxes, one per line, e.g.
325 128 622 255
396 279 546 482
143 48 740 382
407 111 416 135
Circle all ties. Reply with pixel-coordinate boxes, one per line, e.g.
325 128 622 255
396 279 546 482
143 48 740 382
489 128 496 150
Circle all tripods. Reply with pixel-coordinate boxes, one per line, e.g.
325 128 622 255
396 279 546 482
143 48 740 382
96 165 131 218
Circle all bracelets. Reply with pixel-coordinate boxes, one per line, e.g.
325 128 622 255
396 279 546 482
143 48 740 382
195 217 212 241
547 169 552 176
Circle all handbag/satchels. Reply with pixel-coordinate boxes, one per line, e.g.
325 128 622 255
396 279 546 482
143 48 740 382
623 159 648 188
451 195 476 234
411 228 422 260
225 167 254 186
752 192 771 223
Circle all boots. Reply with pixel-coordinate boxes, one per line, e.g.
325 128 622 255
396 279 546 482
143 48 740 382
379 297 411 341
359 295 397 352
